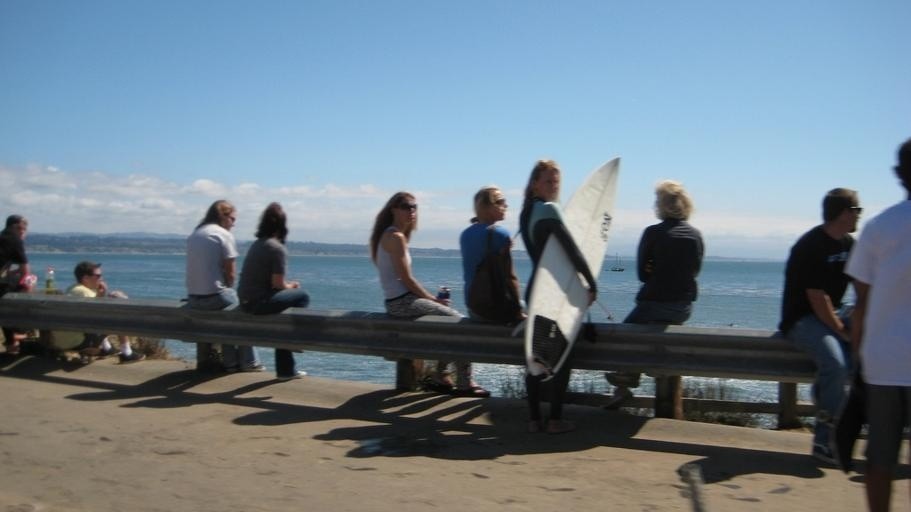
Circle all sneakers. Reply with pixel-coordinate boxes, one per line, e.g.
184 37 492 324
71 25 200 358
222 362 267 373
547 417 578 434
276 368 308 382
810 442 855 470
604 371 640 389
527 419 551 433
118 351 147 364
601 387 634 413
99 347 120 359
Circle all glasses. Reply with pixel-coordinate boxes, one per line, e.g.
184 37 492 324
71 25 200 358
489 198 505 204
844 206 864 215
393 203 418 211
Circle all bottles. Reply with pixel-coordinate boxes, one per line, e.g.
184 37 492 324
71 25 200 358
46 267 53 289
438 287 450 305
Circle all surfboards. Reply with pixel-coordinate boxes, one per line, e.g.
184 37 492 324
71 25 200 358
526 157 622 382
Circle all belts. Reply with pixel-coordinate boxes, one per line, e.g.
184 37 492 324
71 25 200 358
188 293 222 300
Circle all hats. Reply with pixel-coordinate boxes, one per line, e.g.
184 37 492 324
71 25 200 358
74 260 104 282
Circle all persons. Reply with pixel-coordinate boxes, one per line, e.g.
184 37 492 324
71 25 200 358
370 192 491 397
603 181 703 408
237 203 309 381
49 262 146 364
845 138 911 511
186 200 266 372
779 187 868 469
521 161 598 434
460 186 528 323
0 215 37 354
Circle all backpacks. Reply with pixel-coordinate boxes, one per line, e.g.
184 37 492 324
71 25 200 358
463 229 519 329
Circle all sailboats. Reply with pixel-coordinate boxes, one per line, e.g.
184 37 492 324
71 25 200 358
610 251 626 272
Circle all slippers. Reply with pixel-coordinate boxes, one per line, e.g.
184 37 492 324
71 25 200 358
451 383 491 399
423 376 459 394
